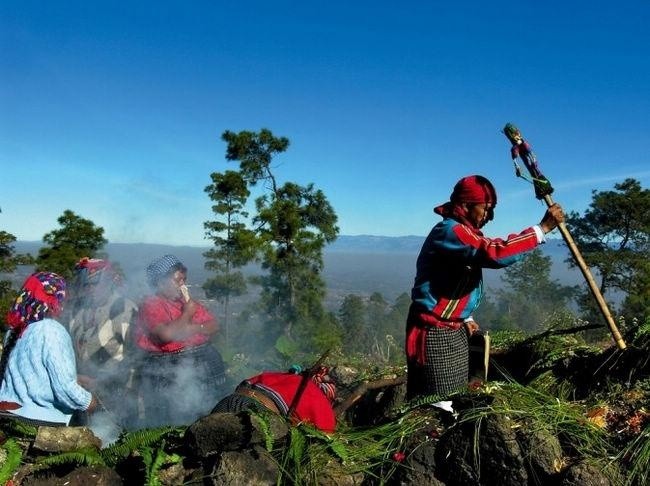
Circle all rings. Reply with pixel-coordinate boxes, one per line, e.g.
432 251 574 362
558 213 564 219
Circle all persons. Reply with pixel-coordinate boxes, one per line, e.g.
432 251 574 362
405 174 568 407
131 252 233 427
208 364 337 436
0 271 100 428
60 252 139 447
